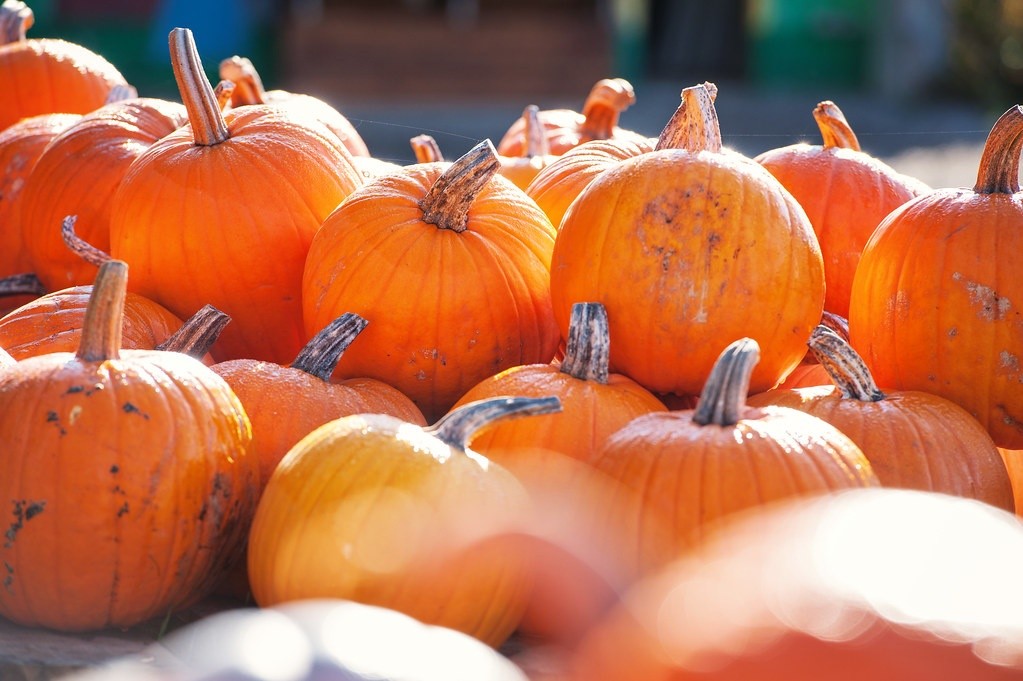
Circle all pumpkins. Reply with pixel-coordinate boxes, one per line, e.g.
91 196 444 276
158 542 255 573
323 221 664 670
3 0 1023 679
